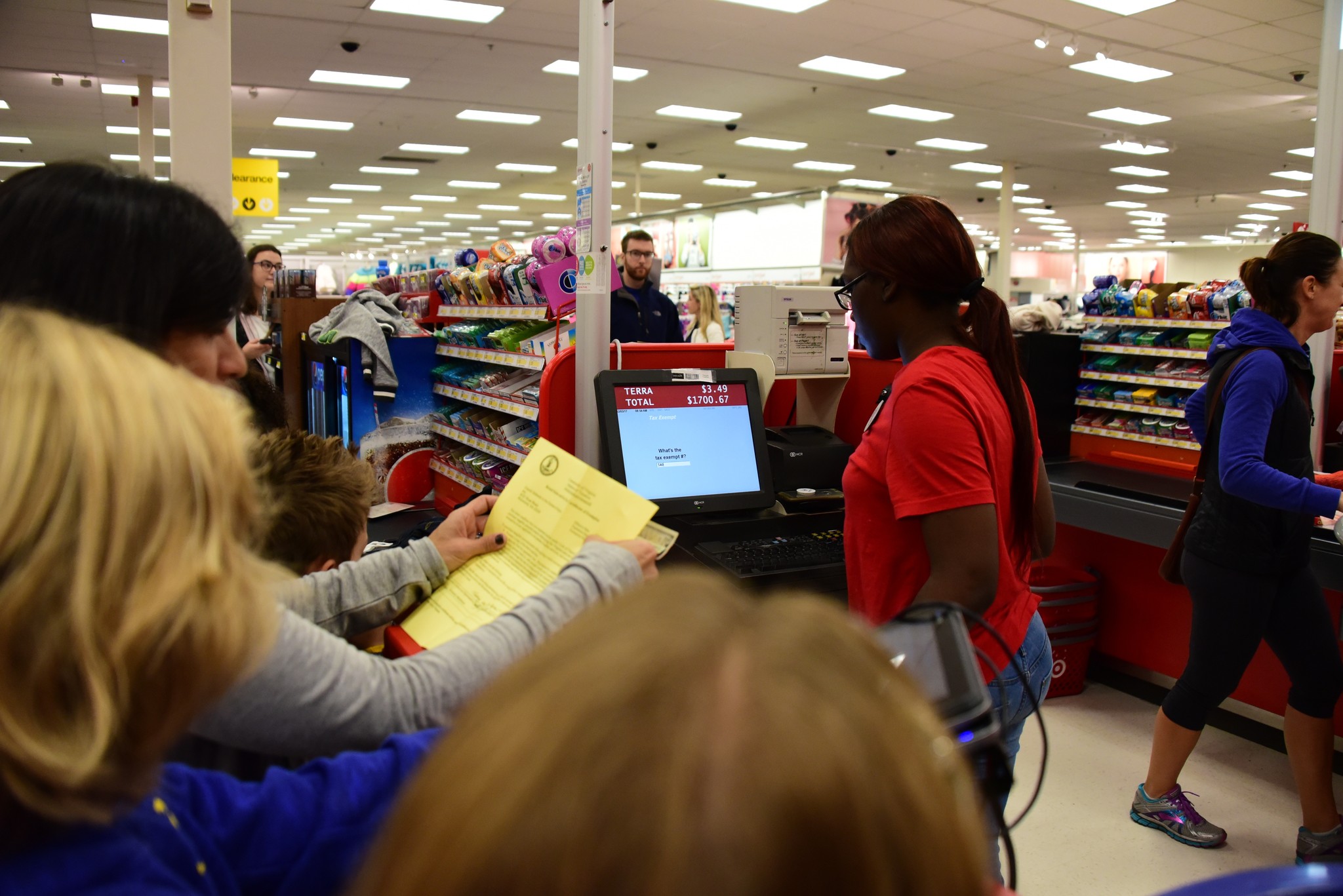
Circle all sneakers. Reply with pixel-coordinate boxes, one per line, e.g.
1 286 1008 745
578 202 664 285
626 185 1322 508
1130 781 1228 848
1295 812 1343 865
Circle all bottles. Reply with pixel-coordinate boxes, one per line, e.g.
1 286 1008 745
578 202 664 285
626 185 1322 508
376 260 390 278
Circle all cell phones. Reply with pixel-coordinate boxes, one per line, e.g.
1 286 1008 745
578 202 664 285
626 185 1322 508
260 338 273 344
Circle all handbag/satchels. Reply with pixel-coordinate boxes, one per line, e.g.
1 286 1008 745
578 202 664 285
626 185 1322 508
1161 492 1203 586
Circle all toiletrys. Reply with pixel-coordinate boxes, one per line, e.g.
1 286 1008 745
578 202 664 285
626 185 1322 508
376 260 389 278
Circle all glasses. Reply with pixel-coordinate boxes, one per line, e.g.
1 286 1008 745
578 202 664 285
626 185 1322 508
253 260 285 272
834 267 877 311
625 249 655 260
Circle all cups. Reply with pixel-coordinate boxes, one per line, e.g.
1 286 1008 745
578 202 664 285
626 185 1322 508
360 423 436 503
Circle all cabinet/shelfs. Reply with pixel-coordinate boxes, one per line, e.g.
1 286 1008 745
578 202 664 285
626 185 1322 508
392 271 579 513
1068 312 1232 479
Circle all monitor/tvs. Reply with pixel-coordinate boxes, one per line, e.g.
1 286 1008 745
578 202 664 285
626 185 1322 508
595 368 776 515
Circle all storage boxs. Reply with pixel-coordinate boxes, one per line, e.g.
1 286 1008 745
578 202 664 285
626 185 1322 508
431 244 622 492
1075 278 1252 441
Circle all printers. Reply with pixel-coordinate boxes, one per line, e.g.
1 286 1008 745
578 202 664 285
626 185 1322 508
734 285 850 375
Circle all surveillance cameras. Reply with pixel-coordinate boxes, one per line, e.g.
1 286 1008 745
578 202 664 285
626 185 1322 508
886 150 896 156
976 198 983 203
718 174 726 178
1045 205 1052 209
725 124 737 130
647 142 657 149
340 41 360 53
1292 74 1306 82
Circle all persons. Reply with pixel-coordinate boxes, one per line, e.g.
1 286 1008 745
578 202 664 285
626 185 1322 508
1127 230 1342 867
833 192 1059 886
840 200 882 266
0 159 998 896
